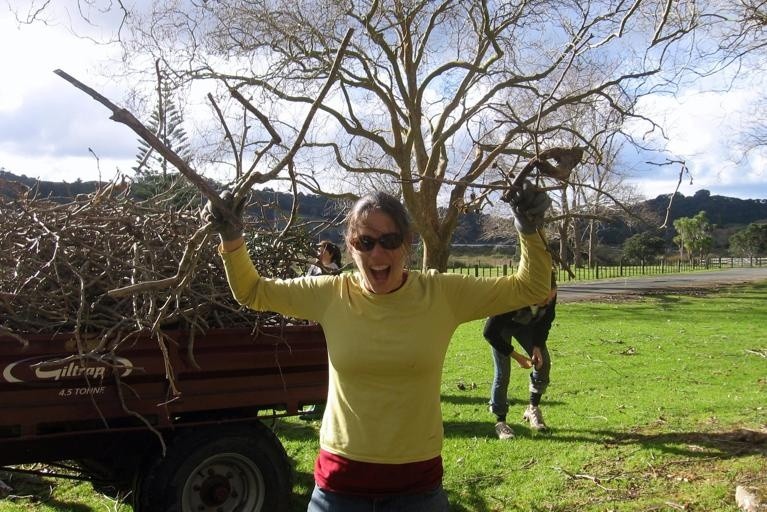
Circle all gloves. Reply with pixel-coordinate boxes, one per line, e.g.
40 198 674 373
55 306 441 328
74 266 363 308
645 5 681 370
198 188 252 242
506 178 551 235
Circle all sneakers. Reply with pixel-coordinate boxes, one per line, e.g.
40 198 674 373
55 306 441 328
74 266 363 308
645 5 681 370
522 406 547 431
494 420 515 440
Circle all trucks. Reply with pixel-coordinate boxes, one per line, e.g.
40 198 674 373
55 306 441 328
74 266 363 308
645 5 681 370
0 324 327 512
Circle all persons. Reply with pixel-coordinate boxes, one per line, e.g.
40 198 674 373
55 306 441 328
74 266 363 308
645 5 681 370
298 237 342 422
201 178 552 512
482 268 559 440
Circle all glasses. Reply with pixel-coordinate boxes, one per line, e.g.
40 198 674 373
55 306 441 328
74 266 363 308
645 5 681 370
349 232 405 252
